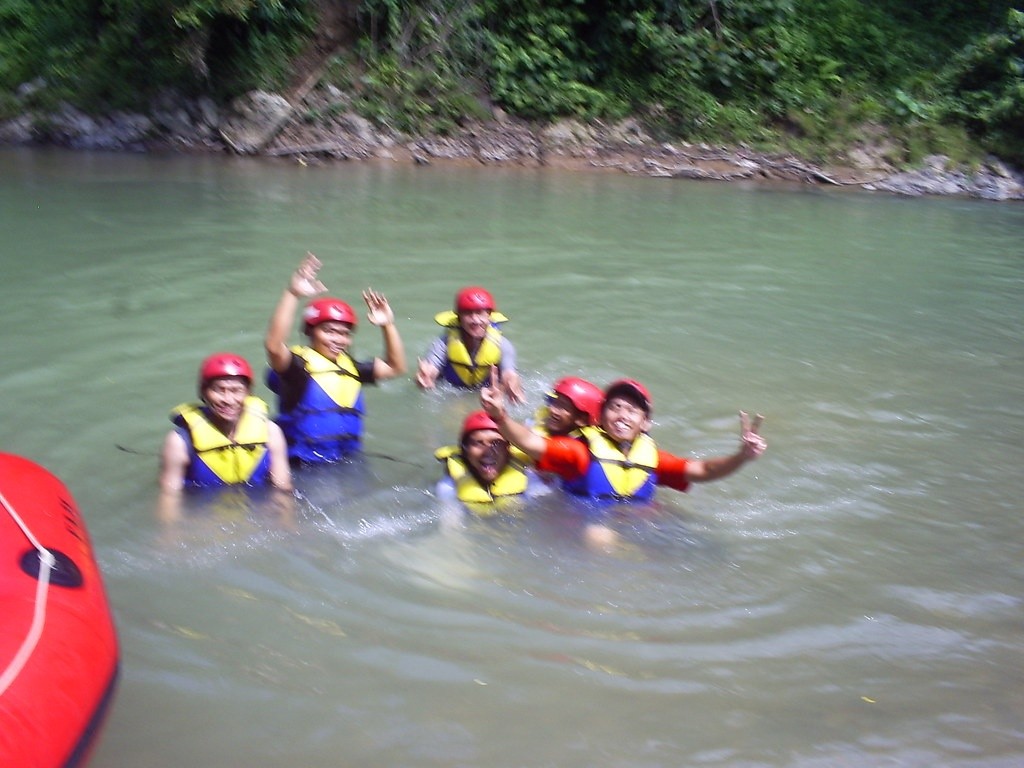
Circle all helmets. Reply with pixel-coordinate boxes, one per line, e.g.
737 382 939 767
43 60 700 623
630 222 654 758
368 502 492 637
303 298 357 336
550 376 606 426
197 353 254 400
456 288 495 310
461 408 502 441
599 380 651 421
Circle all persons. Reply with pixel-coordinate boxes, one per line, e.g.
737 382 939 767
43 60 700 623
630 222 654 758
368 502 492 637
263 252 407 476
157 352 292 509
415 286 768 514
